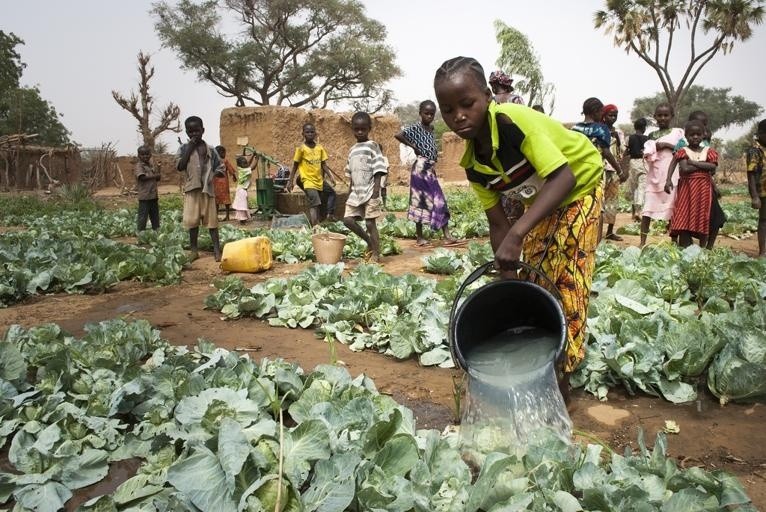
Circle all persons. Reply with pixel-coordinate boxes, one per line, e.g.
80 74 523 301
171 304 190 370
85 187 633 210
343 111 388 266
135 146 162 233
212 146 236 221
532 106 545 114
747 118 766 255
287 123 336 227
296 167 338 222
569 99 728 251
231 149 261 225
174 117 226 262
395 101 469 246
488 71 524 105
433 56 606 413
376 144 390 209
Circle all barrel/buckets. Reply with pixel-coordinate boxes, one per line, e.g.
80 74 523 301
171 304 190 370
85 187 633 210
312 229 347 266
221 236 274 273
447 256 570 402
273 176 290 188
